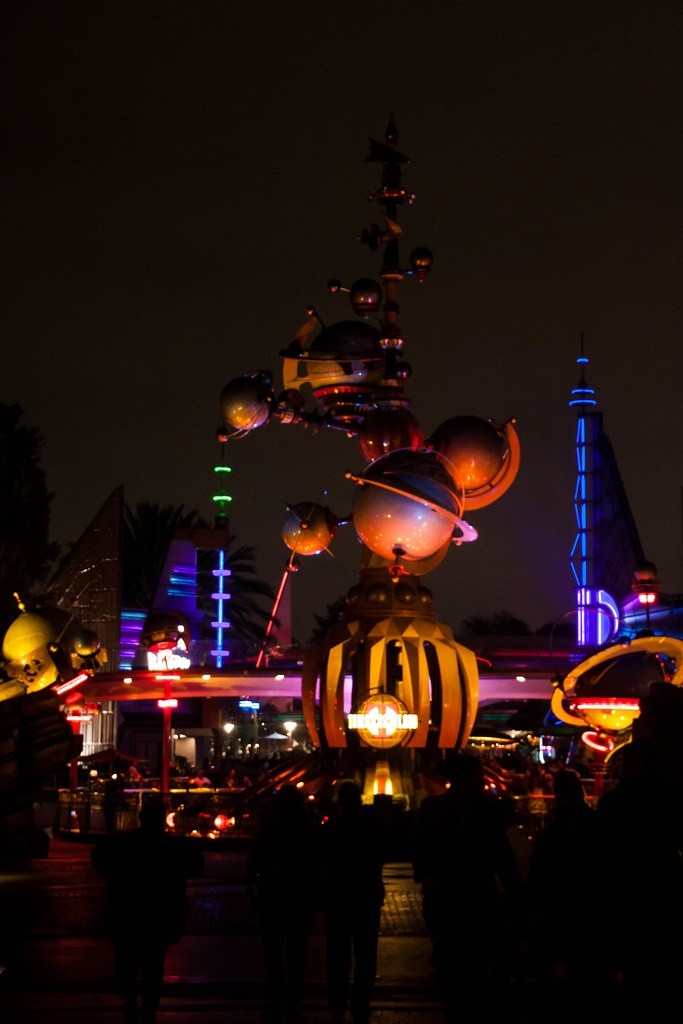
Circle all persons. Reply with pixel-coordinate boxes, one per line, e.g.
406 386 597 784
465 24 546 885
0 681 683 1024
